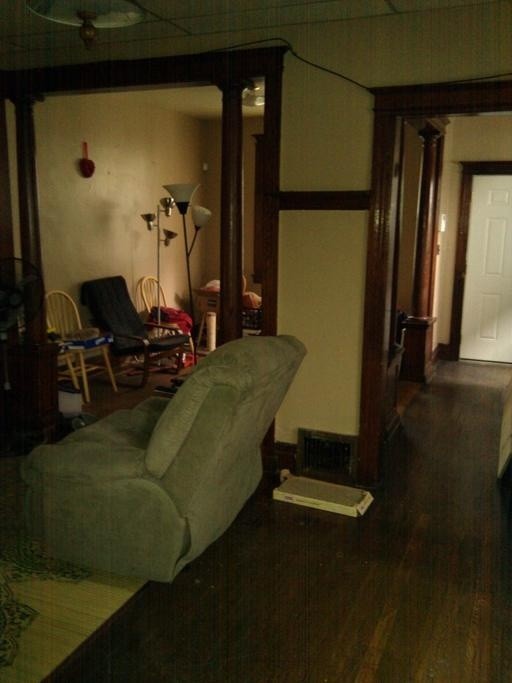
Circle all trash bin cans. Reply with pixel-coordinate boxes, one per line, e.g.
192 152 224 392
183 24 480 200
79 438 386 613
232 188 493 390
400 316 438 384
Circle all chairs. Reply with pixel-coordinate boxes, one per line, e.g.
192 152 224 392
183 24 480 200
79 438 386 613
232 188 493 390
46 275 196 404
17 333 307 586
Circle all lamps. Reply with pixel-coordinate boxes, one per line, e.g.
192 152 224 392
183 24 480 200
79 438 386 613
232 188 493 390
140 181 213 367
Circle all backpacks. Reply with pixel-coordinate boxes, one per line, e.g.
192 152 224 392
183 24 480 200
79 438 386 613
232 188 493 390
150 306 193 336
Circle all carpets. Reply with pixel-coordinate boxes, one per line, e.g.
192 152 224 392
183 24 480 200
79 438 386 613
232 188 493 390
1 451 153 683
